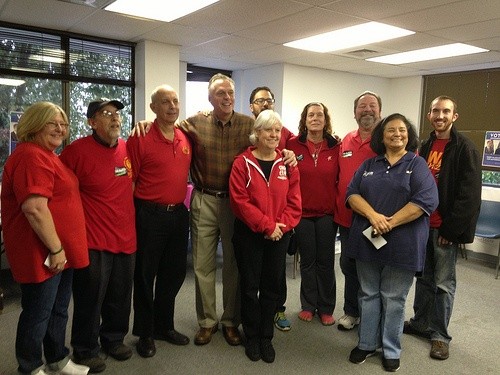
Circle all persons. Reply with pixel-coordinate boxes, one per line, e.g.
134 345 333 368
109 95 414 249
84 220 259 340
0 102 89 375
57 97 137 371
485 141 500 155
402 96 482 360
345 112 439 371
125 84 190 357
129 73 382 346
228 110 302 363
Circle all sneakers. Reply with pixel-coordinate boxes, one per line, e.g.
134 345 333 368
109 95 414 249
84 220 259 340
430 340 449 359
275 312 291 331
31 356 94 375
403 319 431 339
338 314 360 330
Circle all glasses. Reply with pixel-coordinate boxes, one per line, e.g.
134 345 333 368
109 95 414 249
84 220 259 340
252 98 275 105
99 110 121 117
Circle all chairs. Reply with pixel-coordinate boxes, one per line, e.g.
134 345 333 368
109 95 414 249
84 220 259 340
463 200 500 280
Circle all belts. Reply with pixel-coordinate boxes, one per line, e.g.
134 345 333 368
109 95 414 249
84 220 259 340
140 203 184 213
197 186 230 200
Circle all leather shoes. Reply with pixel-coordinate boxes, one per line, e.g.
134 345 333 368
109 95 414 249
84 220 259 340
137 329 190 357
194 323 242 345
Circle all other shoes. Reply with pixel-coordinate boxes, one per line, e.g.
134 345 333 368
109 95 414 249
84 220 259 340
75 342 131 374
349 346 401 371
244 338 275 363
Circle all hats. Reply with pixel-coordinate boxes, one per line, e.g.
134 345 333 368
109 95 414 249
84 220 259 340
87 100 125 117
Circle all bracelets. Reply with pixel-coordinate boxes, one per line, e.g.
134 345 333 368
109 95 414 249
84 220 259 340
51 247 62 255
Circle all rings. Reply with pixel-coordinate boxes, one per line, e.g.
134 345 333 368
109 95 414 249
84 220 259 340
64 260 67 263
